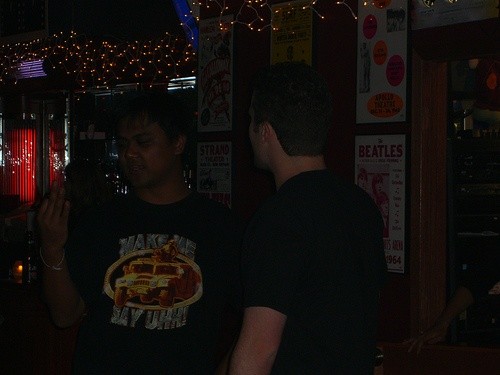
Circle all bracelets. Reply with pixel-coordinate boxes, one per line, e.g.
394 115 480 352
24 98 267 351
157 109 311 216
39 240 66 271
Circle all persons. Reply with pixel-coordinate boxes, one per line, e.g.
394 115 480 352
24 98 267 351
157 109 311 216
36 93 246 375
226 63 387 375
402 240 500 354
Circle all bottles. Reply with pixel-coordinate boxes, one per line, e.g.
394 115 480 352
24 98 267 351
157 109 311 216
21 230 34 288
182 164 194 194
451 100 474 138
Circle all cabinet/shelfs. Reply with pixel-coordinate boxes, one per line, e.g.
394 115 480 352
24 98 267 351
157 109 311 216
405 0 500 354
